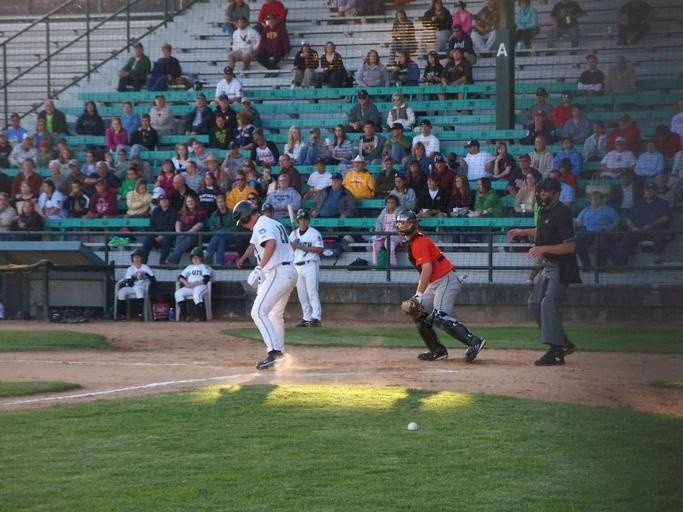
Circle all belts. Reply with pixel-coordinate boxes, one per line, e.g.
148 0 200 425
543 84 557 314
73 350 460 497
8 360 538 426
282 261 291 265
295 260 310 266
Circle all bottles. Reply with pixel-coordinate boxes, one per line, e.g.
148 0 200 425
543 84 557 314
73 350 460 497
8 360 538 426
168 307 174 321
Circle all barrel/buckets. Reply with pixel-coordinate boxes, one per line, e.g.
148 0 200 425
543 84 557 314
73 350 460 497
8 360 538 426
151 292 171 321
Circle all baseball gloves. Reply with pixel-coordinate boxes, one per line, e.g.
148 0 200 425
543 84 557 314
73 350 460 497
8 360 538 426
401 297 430 323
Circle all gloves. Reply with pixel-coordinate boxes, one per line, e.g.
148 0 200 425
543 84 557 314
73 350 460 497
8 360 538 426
247 266 265 288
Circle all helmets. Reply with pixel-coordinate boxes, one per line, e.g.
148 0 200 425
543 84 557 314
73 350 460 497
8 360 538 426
232 199 258 227
262 202 273 212
190 246 203 256
296 209 310 220
395 209 417 223
130 249 146 264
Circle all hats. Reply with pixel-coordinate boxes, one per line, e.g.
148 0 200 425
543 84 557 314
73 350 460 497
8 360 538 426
420 118 432 128
330 172 343 180
203 155 221 164
357 89 368 96
534 87 548 117
615 112 631 145
241 96 250 103
391 122 403 129
464 139 478 148
541 177 561 194
151 187 166 199
351 154 365 162
224 67 232 72
309 126 321 135
433 154 444 163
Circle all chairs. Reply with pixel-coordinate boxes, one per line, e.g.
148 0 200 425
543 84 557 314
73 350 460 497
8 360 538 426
174 265 214 321
114 278 152 322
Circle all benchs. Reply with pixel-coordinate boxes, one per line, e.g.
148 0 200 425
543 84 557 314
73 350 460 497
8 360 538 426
0 0 683 283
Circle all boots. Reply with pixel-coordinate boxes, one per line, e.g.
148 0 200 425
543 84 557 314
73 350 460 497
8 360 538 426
178 300 191 322
196 302 205 321
118 300 128 322
135 298 145 321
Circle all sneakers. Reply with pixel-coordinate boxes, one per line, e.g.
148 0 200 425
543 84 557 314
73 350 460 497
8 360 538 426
418 345 449 361
296 320 311 328
310 320 321 327
463 335 487 363
561 337 576 355
536 350 564 366
255 350 286 370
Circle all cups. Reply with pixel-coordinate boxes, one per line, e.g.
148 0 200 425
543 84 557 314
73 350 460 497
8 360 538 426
324 138 330 147
452 208 458 216
519 203 526 213
565 16 570 24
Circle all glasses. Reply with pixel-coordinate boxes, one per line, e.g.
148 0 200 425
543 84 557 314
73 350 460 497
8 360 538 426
358 96 365 99
225 72 231 75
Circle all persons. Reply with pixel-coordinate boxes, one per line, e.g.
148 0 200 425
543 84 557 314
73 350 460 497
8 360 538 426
546 0 583 56
386 93 415 132
290 41 345 87
118 250 157 321
507 177 583 366
174 246 211 322
224 0 291 79
142 122 474 268
117 43 182 92
464 86 683 267
619 0 654 44
576 54 638 95
395 209 487 362
288 209 325 328
0 100 158 241
185 66 262 150
326 0 354 25
515 0 539 49
356 0 496 116
344 89 381 133
233 200 298 370
149 95 176 135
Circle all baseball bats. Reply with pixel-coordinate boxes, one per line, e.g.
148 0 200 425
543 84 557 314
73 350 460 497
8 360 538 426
287 204 299 245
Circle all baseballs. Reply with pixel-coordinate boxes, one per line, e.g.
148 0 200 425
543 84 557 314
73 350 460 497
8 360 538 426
407 422 418 431
